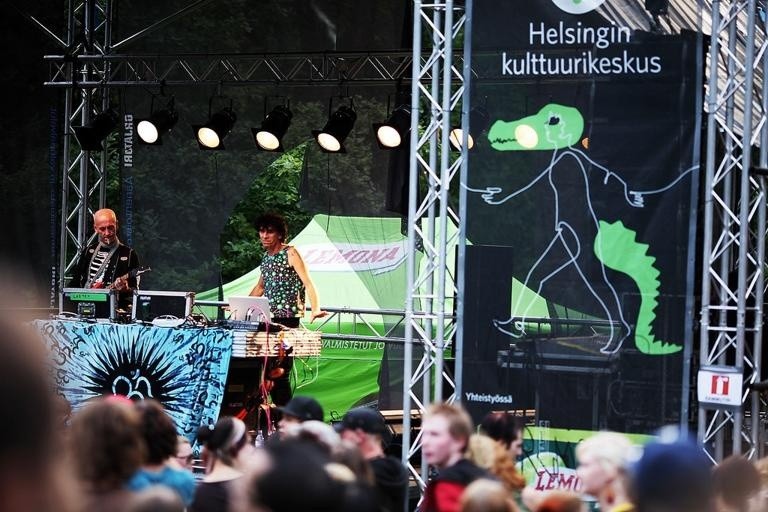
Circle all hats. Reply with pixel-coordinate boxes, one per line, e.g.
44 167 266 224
333 407 386 434
273 395 324 422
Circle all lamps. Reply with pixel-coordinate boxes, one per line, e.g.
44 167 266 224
71 79 495 156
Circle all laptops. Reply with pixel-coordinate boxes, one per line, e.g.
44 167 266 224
230 295 273 323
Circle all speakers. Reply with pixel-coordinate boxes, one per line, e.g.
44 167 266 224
454 244 514 360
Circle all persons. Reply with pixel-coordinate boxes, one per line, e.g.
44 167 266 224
62 208 138 320
249 214 328 405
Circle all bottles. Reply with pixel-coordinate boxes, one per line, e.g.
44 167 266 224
109 288 118 322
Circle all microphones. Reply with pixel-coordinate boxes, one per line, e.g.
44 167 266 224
81 241 89 251
